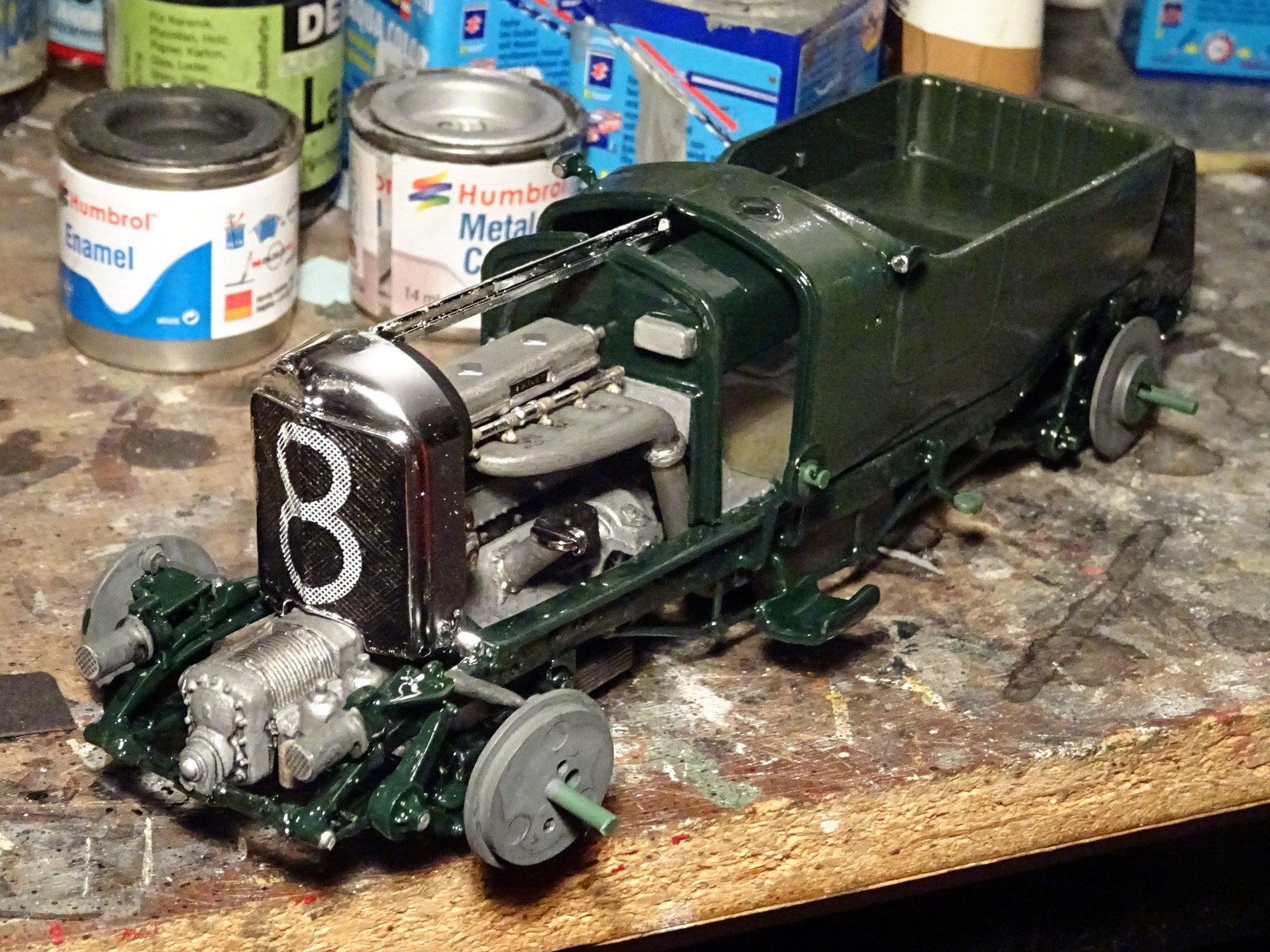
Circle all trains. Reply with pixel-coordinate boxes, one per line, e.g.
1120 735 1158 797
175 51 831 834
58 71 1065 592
76 73 1200 871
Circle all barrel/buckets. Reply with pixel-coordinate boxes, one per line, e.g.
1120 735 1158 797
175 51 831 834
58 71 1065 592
58 84 306 373
344 65 590 341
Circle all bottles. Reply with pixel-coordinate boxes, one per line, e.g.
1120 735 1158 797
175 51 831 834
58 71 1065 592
0 0 343 231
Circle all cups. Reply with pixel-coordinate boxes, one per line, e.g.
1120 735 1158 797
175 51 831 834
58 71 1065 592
880 2 1047 103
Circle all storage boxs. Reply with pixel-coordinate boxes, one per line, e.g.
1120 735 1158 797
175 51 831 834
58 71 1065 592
1098 0 1270 87
342 1 888 179
